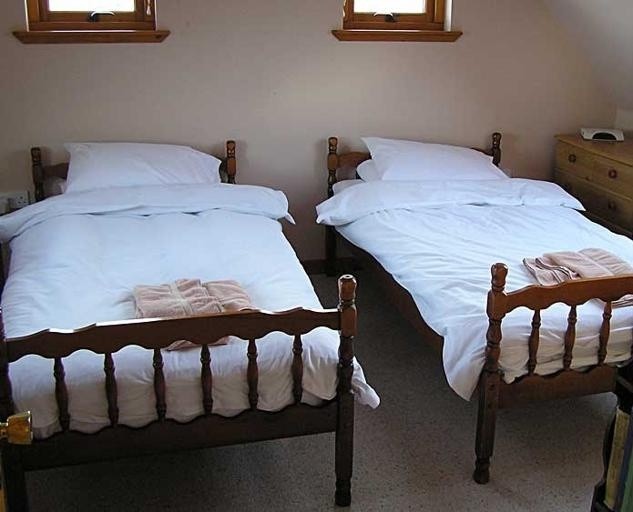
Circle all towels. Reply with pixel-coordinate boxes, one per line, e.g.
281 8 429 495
523 248 633 309
133 275 254 351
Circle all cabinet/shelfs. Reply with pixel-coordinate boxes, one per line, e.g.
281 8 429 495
552 133 633 240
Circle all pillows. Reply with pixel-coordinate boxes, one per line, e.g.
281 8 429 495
357 135 510 186
60 139 224 191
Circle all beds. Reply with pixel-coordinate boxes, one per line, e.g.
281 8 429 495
325 133 633 485
1 138 358 507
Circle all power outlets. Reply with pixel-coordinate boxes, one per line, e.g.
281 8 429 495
0 191 30 210
